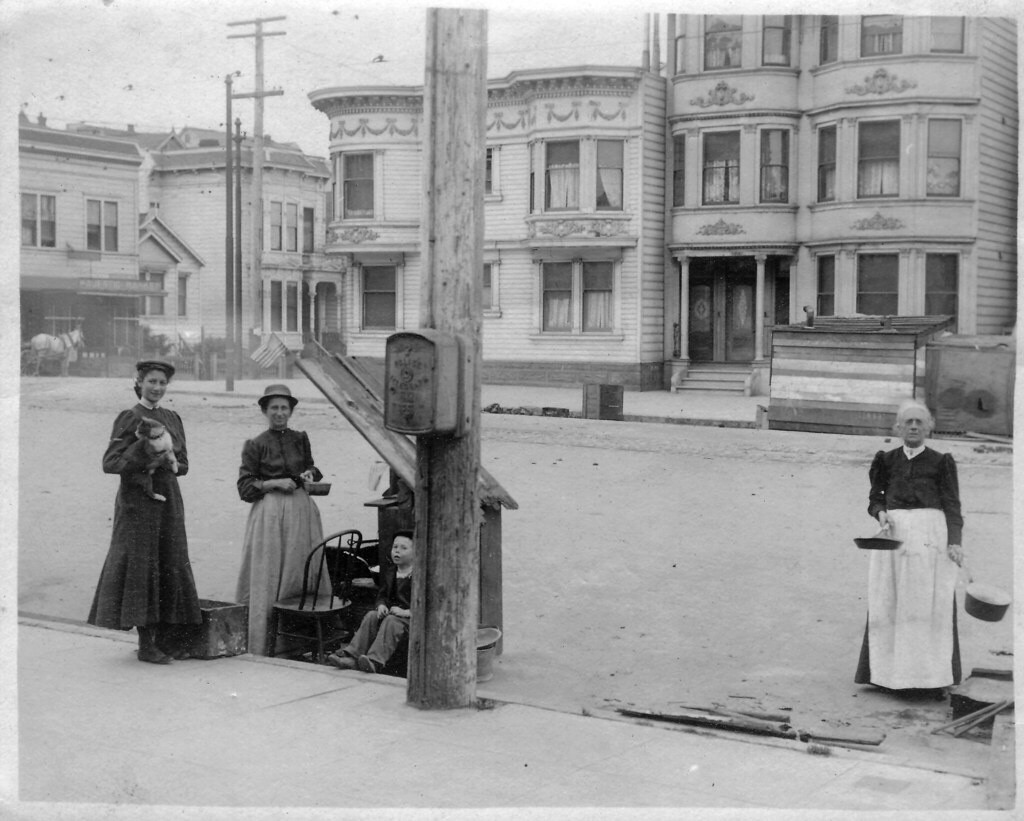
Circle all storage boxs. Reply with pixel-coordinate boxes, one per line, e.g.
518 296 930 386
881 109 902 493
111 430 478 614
155 598 249 658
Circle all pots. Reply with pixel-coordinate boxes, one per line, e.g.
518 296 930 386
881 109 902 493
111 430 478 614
854 522 903 550
961 561 1011 621
345 551 379 584
303 476 332 496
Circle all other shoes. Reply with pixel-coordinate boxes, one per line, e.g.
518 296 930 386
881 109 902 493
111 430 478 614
138 643 174 663
328 654 355 672
934 687 949 701
878 686 895 693
359 653 376 673
153 641 190 660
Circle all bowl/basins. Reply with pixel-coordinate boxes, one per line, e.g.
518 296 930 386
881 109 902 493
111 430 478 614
477 628 502 650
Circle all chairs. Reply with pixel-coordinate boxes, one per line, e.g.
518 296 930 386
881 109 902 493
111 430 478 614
269 528 362 666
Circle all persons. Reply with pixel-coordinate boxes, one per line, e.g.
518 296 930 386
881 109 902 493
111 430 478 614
855 399 964 702
238 385 332 654
329 529 416 672
87 359 203 663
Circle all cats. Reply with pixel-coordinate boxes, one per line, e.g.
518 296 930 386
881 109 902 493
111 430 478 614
121 416 179 502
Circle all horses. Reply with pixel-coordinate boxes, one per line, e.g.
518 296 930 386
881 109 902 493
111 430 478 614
31 329 86 377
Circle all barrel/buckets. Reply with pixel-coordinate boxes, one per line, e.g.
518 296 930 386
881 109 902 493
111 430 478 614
477 646 500 682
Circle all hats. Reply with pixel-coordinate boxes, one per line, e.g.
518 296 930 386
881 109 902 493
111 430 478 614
258 385 298 407
136 360 175 377
